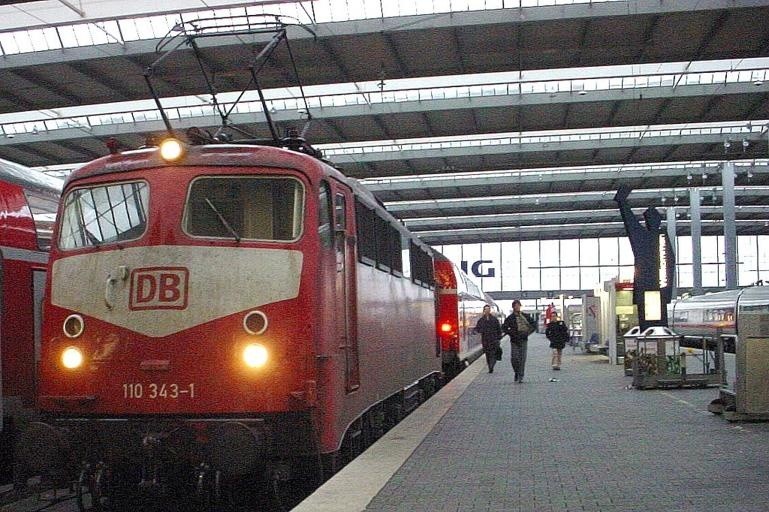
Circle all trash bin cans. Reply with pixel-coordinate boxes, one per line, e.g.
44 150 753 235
637 325 681 376
623 326 641 377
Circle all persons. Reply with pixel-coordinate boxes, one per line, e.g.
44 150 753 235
476 305 502 373
501 300 537 383
546 312 570 370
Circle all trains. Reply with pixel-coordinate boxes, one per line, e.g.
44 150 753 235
668 286 769 352
0 155 141 477
15 126 508 512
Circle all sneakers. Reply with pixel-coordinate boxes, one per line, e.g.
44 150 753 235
513 374 524 386
550 364 565 371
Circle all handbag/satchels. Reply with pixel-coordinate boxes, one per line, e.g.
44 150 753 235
496 348 504 363
